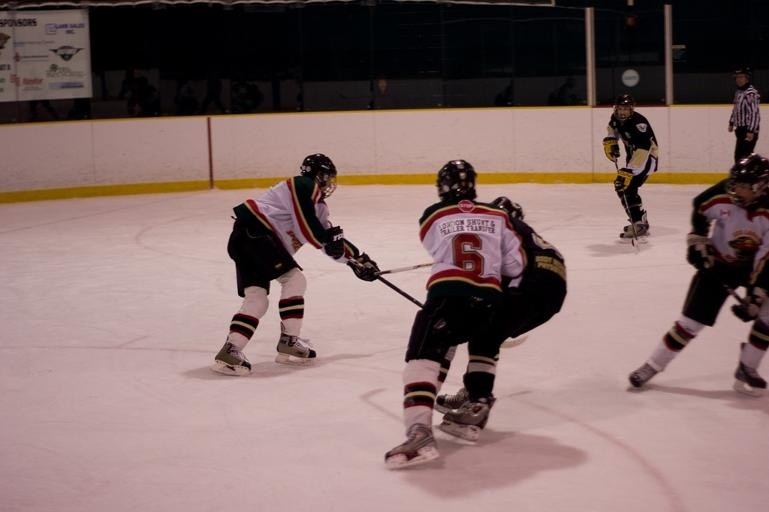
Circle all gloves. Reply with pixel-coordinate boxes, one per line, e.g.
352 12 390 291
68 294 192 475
602 137 620 162
347 252 379 281
324 226 345 261
732 286 767 322
687 233 715 270
614 168 633 191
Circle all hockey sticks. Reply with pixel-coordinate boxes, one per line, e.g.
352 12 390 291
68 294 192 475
616 162 639 247
345 254 528 348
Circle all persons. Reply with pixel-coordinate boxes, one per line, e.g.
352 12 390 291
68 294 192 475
436 195 567 410
30 42 769 120
627 152 769 387
384 157 529 466
601 91 662 238
727 65 760 163
213 152 381 372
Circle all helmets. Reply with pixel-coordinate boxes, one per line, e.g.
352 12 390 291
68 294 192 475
732 66 752 81
612 93 633 120
490 196 524 221
300 153 336 199
437 159 475 195
725 153 769 207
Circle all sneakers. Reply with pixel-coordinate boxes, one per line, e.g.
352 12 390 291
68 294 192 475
443 393 496 429
620 209 649 237
384 424 435 460
734 361 767 389
277 333 316 358
436 387 469 409
629 363 657 387
215 342 251 371
